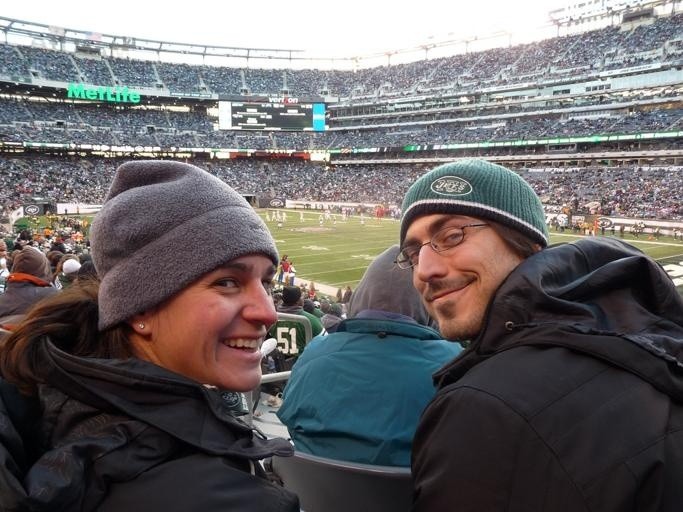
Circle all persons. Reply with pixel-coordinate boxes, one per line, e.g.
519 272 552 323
275 245 466 470
0 9 683 400
0 161 299 512
395 158 683 511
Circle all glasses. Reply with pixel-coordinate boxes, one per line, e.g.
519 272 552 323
392 220 487 271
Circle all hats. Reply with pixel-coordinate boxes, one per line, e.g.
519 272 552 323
88 160 280 331
281 284 343 316
11 246 54 284
398 156 549 260
345 243 440 330
62 259 83 275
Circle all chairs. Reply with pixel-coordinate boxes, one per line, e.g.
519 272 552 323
270 451 414 512
1 327 12 350
